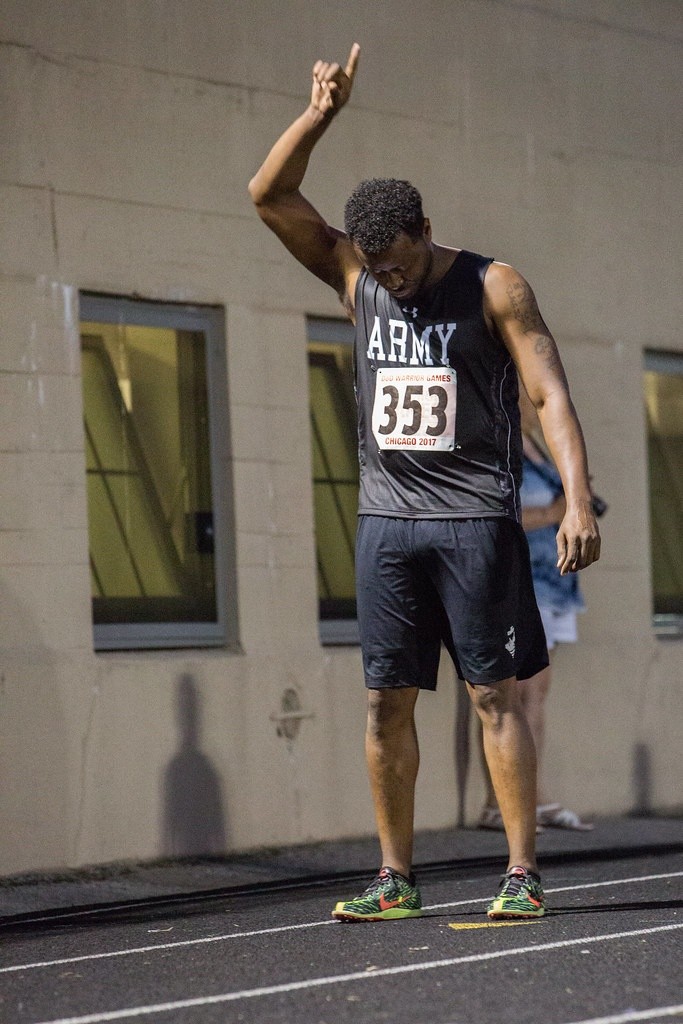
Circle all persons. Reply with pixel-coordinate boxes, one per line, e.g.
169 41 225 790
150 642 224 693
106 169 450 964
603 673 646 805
248 42 601 922
475 378 595 834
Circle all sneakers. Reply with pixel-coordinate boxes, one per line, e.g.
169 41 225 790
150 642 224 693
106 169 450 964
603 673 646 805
486 865 546 918
330 866 423 921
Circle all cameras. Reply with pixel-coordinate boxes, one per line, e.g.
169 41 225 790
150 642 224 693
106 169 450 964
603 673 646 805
591 496 607 517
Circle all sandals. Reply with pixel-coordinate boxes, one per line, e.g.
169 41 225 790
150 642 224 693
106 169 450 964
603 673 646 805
535 799 595 832
479 801 544 835
